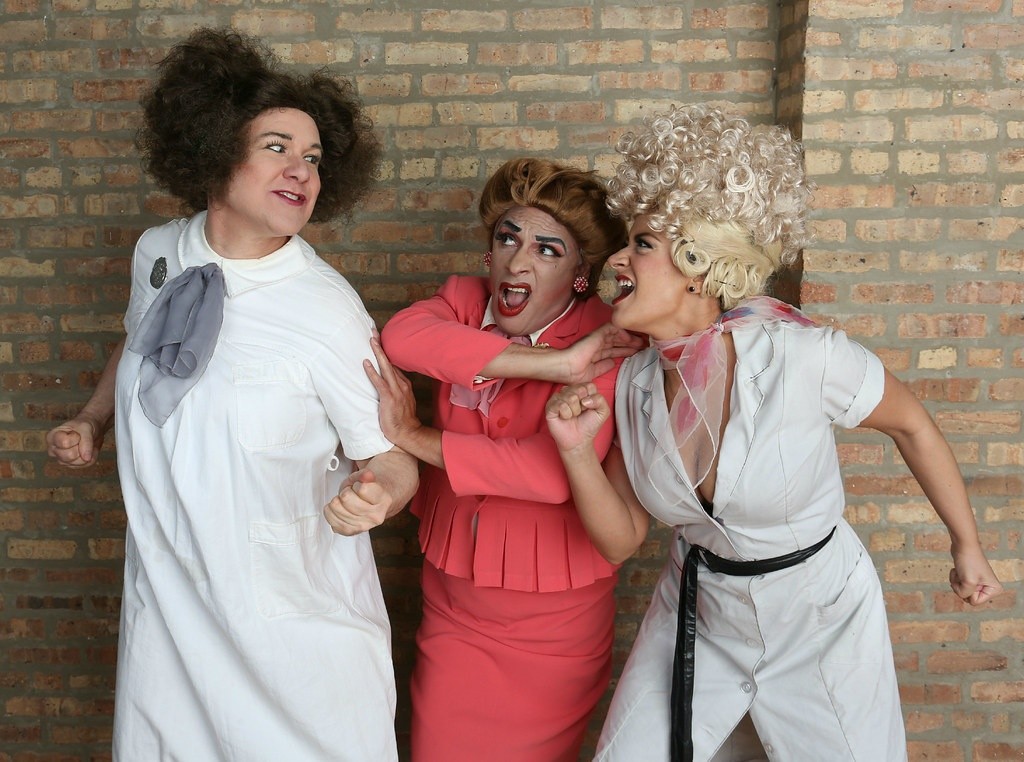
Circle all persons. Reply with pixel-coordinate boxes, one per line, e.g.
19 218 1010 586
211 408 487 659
365 157 650 762
546 104 1001 762
45 27 421 762
